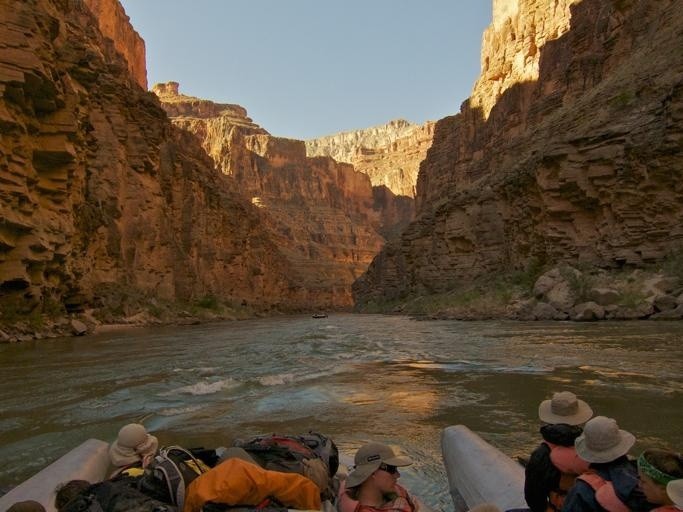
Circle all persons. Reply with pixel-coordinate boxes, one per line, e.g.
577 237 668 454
334 441 419 512
637 449 683 512
524 392 593 511
109 423 159 475
560 415 635 512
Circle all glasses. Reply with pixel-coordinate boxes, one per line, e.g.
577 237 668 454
379 465 397 474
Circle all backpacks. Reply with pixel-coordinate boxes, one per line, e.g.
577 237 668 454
55 431 339 512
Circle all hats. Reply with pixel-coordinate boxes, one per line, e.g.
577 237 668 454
538 391 636 462
666 479 683 508
110 424 159 467
345 442 413 488
